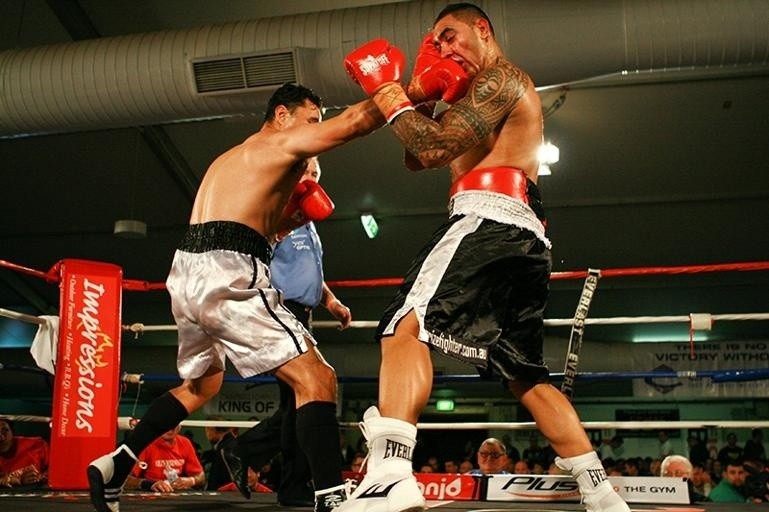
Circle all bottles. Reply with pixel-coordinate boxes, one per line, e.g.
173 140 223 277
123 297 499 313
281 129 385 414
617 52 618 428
164 464 178 485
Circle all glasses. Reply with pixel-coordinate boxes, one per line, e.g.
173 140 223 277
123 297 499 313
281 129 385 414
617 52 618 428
479 450 505 458
662 470 690 477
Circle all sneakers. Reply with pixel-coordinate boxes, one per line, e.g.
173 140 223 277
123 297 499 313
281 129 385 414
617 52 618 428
85 443 143 512
313 476 356 512
220 437 257 499
277 491 316 508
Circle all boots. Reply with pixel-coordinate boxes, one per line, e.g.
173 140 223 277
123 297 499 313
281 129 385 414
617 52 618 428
329 404 426 512
556 453 631 512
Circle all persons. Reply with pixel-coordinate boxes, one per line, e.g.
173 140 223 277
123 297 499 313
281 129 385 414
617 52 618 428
91 4 632 512
0 395 769 512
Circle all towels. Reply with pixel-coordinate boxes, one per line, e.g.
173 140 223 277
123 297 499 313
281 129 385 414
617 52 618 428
28 312 60 376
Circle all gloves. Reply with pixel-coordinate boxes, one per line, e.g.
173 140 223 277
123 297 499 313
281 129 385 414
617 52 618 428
342 38 416 127
274 179 336 243
412 32 442 73
403 58 470 107
